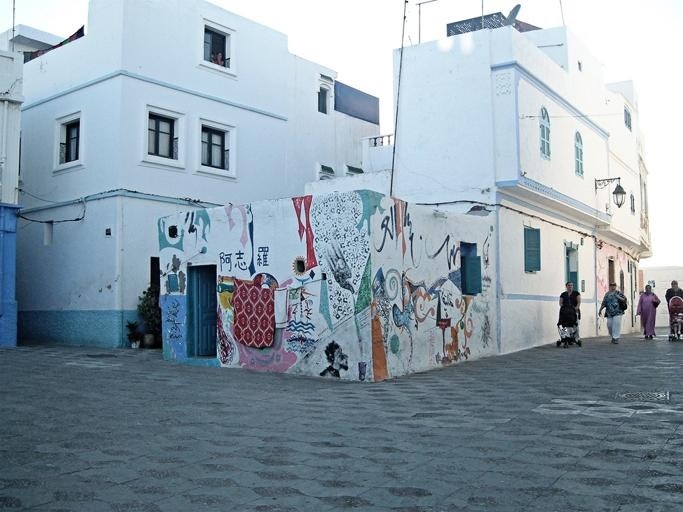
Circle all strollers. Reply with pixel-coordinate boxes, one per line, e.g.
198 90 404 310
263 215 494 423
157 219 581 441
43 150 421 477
668 297 683 342
556 305 582 348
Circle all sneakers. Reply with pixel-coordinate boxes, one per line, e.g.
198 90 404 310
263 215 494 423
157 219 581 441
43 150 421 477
611 338 620 344
645 335 653 339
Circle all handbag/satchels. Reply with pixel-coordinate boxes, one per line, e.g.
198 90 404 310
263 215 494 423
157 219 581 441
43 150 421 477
618 298 627 311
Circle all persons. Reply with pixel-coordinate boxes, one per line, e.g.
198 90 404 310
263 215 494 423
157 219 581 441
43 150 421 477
212 50 226 68
559 281 582 345
636 284 661 340
598 281 628 344
666 278 683 342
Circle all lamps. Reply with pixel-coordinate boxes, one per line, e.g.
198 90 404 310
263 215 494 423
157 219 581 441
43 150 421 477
596 178 626 209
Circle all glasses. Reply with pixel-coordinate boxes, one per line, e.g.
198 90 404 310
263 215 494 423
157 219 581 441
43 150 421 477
609 283 616 286
